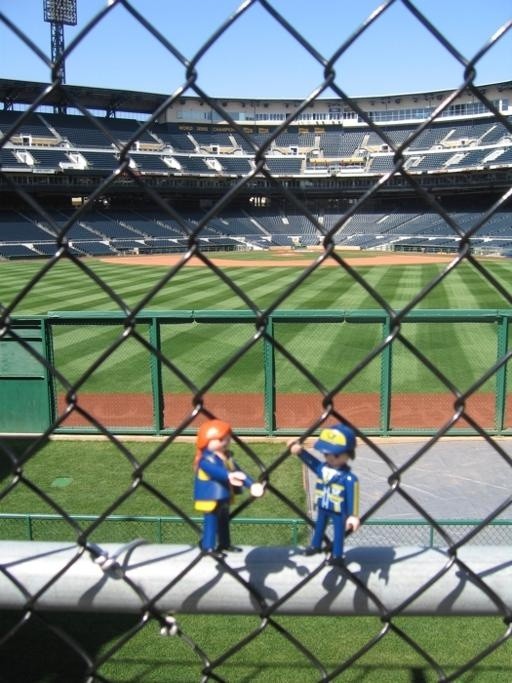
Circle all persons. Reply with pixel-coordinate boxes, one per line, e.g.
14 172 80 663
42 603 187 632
189 417 268 558
288 422 360 566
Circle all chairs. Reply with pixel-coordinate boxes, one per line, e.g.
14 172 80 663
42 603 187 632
1 122 512 261
